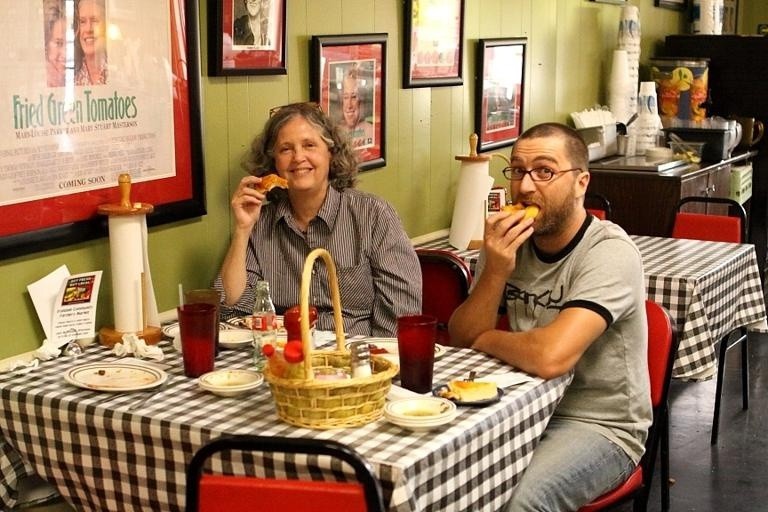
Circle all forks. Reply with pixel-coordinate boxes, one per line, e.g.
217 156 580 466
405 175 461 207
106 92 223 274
462 371 476 384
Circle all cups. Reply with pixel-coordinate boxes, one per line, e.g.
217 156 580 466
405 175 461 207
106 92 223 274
608 3 659 155
667 115 764 158
184 289 220 358
397 313 435 392
692 0 725 34
177 302 217 377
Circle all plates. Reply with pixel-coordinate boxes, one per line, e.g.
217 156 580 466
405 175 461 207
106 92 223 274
346 337 449 361
382 396 457 430
161 314 286 353
198 369 264 396
67 362 169 392
431 382 504 406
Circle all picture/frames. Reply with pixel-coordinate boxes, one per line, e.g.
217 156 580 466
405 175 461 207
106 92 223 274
685 0 739 35
475 37 527 154
654 0 684 12
0 0 209 260
208 1 287 77
403 1 465 89
312 32 389 175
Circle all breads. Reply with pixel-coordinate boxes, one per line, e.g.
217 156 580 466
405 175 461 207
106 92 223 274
443 378 498 403
252 173 288 194
498 202 540 228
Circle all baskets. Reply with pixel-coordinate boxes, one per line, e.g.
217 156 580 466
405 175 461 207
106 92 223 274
263 249 400 430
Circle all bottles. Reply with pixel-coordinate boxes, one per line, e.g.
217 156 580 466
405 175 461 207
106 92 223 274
283 340 305 380
351 340 373 380
282 307 319 353
263 345 286 374
251 281 277 368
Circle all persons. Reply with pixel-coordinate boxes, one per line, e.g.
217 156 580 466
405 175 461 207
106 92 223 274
70 1 111 91
233 1 275 47
42 1 68 93
440 122 657 511
207 99 427 340
333 64 375 148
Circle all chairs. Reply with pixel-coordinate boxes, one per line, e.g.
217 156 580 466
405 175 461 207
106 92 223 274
666 196 750 445
584 192 613 222
414 248 473 346
577 298 678 512
186 433 387 512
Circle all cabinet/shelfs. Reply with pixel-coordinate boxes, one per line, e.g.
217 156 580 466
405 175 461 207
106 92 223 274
663 34 768 293
585 148 762 238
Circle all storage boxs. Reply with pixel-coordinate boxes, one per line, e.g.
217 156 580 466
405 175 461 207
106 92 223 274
660 127 729 164
729 161 753 205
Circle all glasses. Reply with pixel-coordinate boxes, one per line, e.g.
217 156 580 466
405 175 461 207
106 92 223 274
269 101 324 119
502 166 583 182
